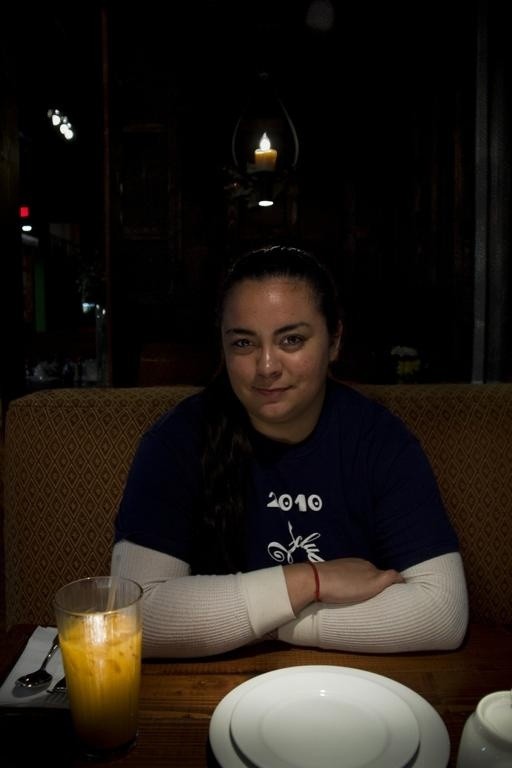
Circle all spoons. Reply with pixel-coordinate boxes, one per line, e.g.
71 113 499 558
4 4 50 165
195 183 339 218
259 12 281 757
14 634 60 689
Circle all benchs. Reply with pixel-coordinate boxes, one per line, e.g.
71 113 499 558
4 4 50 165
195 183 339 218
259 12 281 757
1 385 512 623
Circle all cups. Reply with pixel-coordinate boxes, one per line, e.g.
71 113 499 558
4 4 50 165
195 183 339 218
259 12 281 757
51 576 144 761
453 690 512 768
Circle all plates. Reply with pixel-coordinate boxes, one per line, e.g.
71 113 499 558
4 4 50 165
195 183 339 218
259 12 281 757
208 664 451 768
229 668 421 768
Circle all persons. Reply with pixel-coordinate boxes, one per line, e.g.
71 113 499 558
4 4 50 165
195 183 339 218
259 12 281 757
108 246 472 662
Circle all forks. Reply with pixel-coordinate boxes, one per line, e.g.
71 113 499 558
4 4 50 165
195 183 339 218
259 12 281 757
45 675 69 696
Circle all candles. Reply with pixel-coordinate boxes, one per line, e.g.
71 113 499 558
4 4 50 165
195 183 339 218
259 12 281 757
250 121 281 172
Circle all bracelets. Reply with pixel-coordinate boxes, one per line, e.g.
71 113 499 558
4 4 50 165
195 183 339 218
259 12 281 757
305 557 322 601
